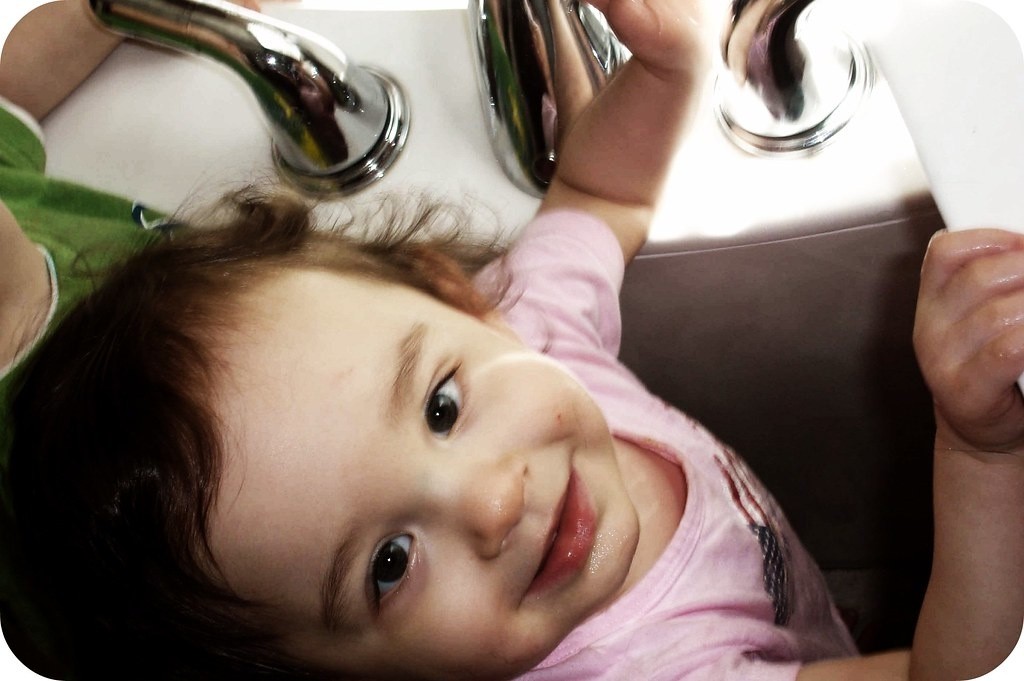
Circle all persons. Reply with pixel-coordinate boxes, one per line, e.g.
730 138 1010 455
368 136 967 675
0 0 1024 681
1 0 264 392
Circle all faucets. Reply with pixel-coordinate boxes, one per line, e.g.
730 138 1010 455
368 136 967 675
466 0 632 198
